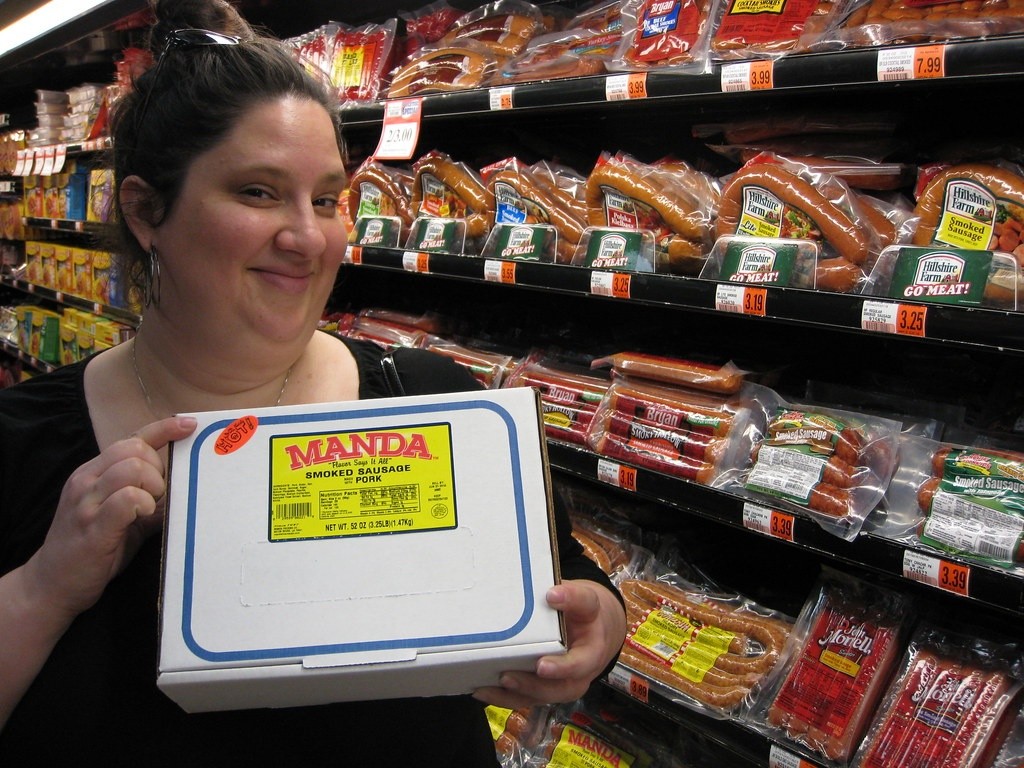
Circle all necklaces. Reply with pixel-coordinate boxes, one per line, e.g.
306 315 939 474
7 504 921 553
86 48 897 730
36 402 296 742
133 323 294 421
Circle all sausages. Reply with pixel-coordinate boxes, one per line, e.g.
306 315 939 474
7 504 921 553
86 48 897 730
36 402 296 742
348 0 1024 768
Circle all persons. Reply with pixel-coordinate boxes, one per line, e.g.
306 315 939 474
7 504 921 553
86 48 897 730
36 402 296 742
0 0 626 768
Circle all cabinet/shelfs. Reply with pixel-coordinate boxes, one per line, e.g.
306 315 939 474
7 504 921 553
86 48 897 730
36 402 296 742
0 2 1023 766
